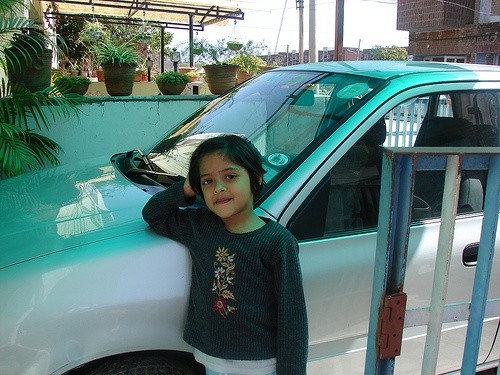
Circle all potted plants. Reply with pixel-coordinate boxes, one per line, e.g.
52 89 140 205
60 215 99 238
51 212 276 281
226 40 243 51
203 63 239 95
4 34 146 98
223 50 267 84
154 71 189 95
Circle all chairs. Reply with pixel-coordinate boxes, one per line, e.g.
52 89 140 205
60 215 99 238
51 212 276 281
300 98 386 231
412 117 498 219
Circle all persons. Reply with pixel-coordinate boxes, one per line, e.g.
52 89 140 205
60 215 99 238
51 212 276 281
142 135 309 375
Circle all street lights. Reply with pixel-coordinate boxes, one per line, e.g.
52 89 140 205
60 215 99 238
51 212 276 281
170 47 180 72
145 57 154 82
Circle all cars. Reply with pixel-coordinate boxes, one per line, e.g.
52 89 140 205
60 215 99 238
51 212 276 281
1 59 500 375
